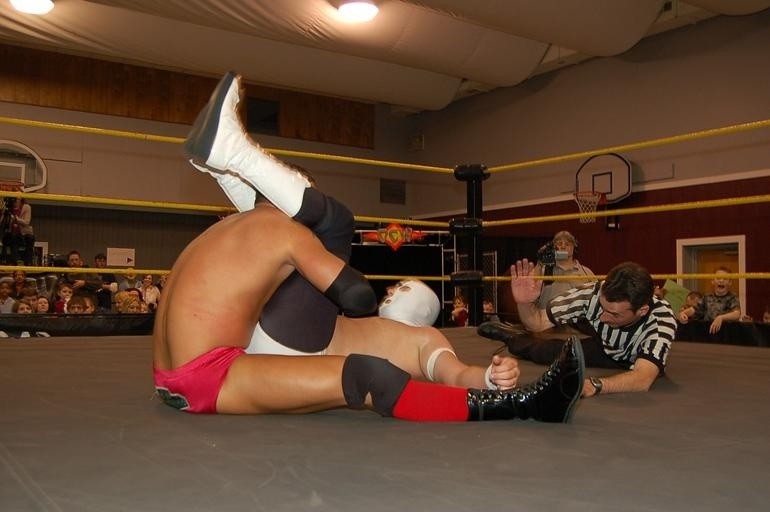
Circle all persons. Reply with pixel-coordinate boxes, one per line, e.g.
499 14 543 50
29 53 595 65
483 301 501 322
138 300 148 313
655 285 668 299
115 291 130 313
127 288 143 301
92 253 119 314
1 276 16 313
119 267 142 290
139 274 161 313
685 291 703 308
179 69 521 391
679 266 741 334
151 164 585 422
9 269 26 300
18 286 40 312
55 283 73 313
741 315 753 322
452 295 469 327
61 250 103 297
476 259 678 398
532 230 598 310
3 197 36 266
81 294 95 315
121 299 142 313
36 296 50 313
67 297 87 315
12 299 35 314
155 273 169 292
762 307 770 322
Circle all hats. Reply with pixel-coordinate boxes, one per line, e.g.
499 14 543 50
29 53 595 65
95 253 106 259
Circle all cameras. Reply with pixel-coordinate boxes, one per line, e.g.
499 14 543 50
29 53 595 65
540 241 570 266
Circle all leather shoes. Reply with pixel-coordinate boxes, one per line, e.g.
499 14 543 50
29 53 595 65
477 321 527 341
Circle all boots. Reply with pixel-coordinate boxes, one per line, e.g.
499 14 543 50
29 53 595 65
467 335 584 423
181 104 257 214
193 70 310 217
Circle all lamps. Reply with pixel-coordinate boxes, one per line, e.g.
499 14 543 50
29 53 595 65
397 134 427 154
335 1 379 25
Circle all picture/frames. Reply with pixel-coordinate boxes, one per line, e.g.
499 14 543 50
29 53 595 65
378 177 408 205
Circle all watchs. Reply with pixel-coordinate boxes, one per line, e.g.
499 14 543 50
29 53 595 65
589 376 603 397
